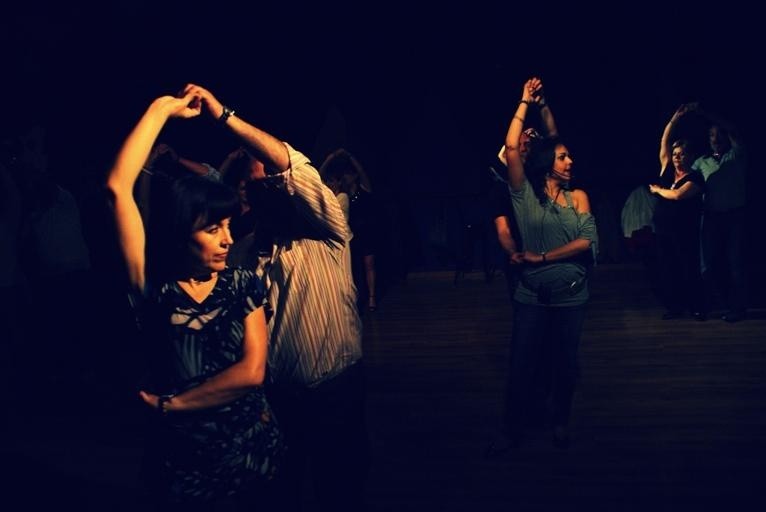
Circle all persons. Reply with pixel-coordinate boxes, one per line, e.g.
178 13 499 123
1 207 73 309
105 87 280 512
503 79 597 436
690 101 748 321
184 83 370 512
318 144 377 311
136 139 268 236
493 78 559 381
648 104 706 321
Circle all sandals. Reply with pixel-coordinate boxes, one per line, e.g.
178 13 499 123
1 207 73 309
368 294 377 312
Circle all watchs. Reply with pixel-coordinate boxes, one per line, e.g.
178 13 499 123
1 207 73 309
219 105 234 122
158 393 173 411
540 251 545 262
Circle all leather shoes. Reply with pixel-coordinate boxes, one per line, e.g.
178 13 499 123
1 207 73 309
663 311 686 320
723 310 746 322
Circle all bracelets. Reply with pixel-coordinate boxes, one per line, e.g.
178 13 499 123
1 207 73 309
517 99 530 105
